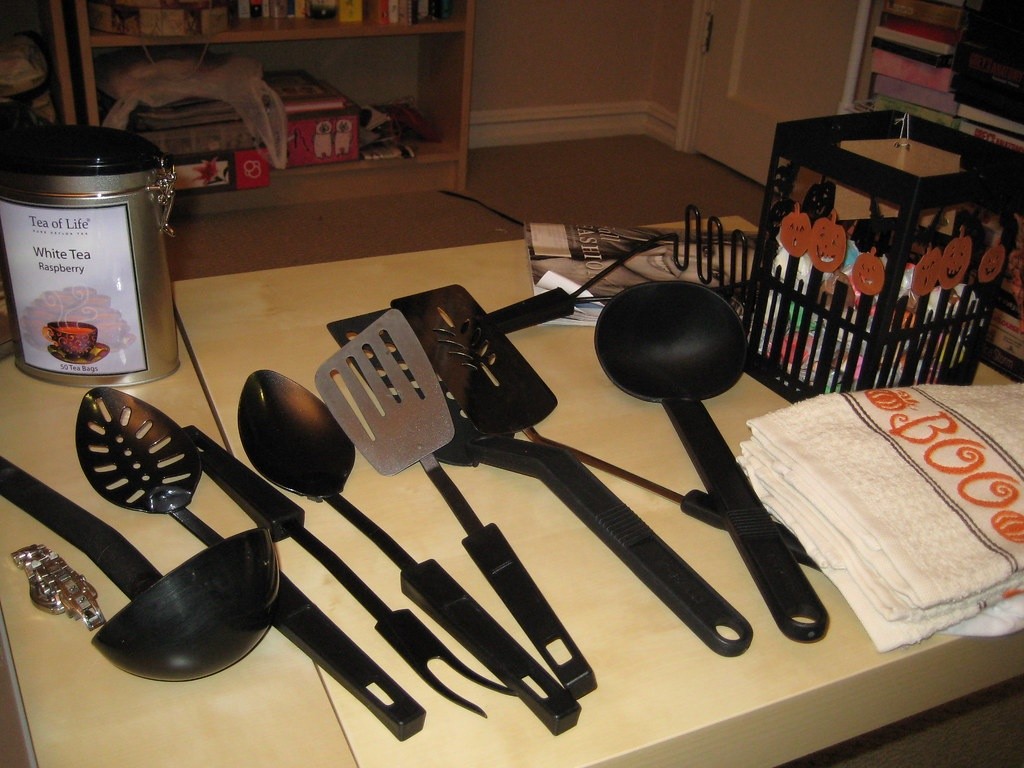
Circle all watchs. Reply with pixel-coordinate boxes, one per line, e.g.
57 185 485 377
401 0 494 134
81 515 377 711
11 543 105 631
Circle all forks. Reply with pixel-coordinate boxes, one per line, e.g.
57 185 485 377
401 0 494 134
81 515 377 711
181 425 517 717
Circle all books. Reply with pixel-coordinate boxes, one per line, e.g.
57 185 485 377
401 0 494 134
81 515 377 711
523 218 757 328
868 0 1024 380
236 0 449 26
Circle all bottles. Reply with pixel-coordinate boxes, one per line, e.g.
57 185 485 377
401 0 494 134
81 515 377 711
2 125 179 385
248 0 262 19
311 0 336 19
429 0 454 19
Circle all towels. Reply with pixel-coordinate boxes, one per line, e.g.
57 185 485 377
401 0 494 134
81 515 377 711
733 381 1024 654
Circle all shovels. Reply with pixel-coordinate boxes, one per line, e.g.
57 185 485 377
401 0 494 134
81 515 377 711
326 282 827 658
313 306 600 702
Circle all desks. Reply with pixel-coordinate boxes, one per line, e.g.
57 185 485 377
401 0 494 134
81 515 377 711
1 215 1024 768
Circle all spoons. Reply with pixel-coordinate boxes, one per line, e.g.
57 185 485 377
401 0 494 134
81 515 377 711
239 370 578 735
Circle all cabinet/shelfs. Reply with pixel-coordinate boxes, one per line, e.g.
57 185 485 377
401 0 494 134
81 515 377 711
37 0 477 213
852 0 1024 157
